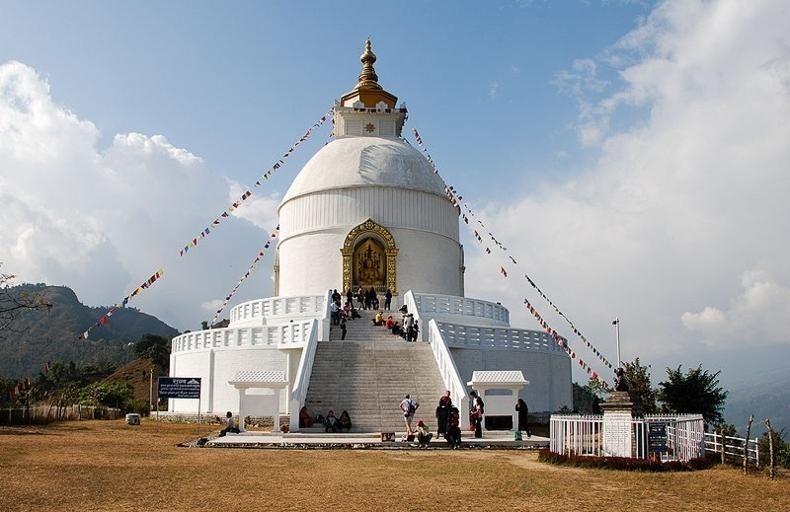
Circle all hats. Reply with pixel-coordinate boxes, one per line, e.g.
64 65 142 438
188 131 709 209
416 420 425 426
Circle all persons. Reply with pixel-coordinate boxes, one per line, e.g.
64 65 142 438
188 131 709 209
413 418 434 448
515 398 531 439
336 410 352 430
355 257 386 291
435 390 486 448
219 411 235 437
328 285 421 343
322 410 339 433
300 405 315 428
399 394 420 435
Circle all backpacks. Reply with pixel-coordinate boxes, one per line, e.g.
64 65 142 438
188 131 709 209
406 400 415 413
